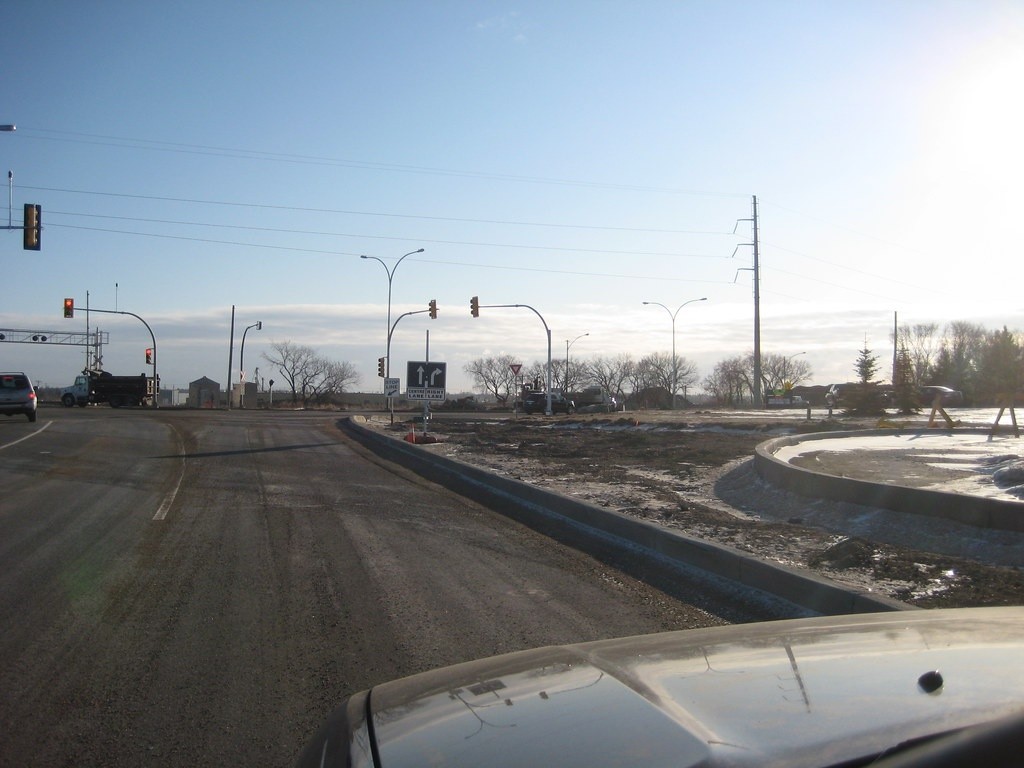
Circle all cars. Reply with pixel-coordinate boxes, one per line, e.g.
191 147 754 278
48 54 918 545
919 385 963 408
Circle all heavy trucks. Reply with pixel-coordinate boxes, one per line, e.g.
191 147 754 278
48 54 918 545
767 395 810 408
61 375 147 408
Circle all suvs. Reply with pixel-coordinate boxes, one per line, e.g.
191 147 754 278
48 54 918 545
0 371 39 422
525 393 577 414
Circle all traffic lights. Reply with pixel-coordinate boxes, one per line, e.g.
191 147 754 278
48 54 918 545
146 348 151 363
429 299 436 319
470 296 479 317
23 204 42 251
64 298 73 318
378 357 385 378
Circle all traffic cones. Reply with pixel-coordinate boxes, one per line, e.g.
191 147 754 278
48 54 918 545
408 422 416 442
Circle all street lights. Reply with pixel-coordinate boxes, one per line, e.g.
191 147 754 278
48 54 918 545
240 321 262 408
784 352 807 383
361 248 425 408
643 298 707 411
566 333 590 390
